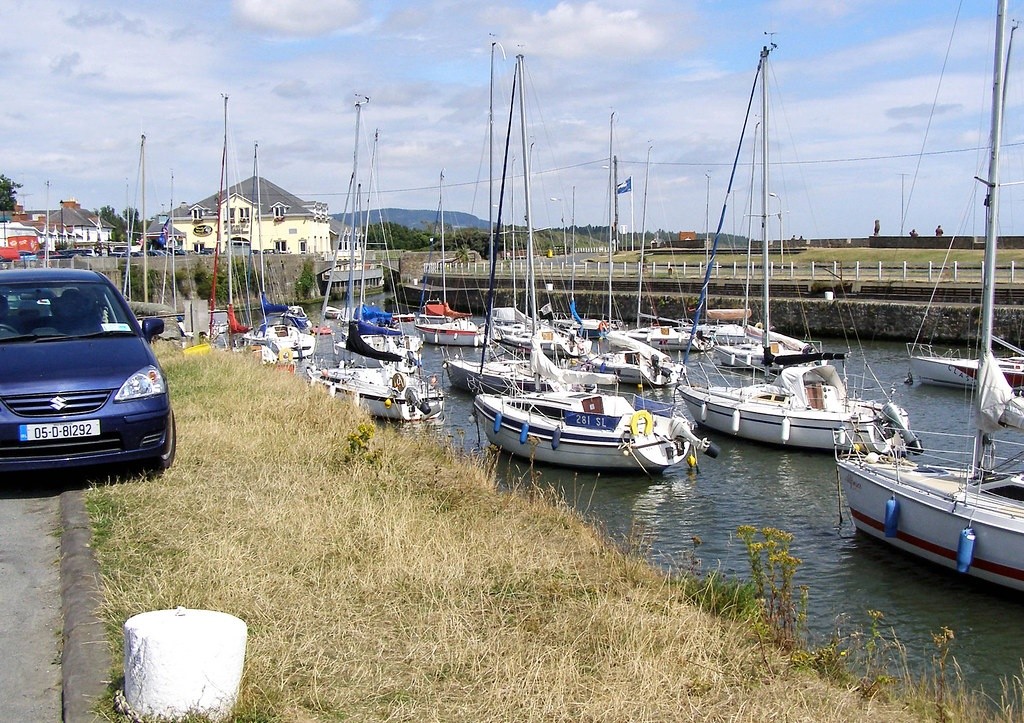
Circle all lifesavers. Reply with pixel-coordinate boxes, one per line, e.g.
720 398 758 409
755 322 763 329
392 373 405 390
278 348 293 362
632 410 655 438
599 321 607 332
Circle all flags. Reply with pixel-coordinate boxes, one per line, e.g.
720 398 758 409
614 176 631 195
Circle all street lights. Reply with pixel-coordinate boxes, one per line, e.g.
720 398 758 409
493 204 506 261
770 192 784 270
550 197 567 263
732 188 737 248
704 169 711 273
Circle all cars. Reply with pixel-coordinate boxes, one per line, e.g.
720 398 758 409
0 268 178 475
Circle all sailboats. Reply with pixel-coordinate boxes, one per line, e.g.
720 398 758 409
0 28 828 423
902 21 1024 402
834 1 1024 600
473 44 724 475
675 44 911 459
